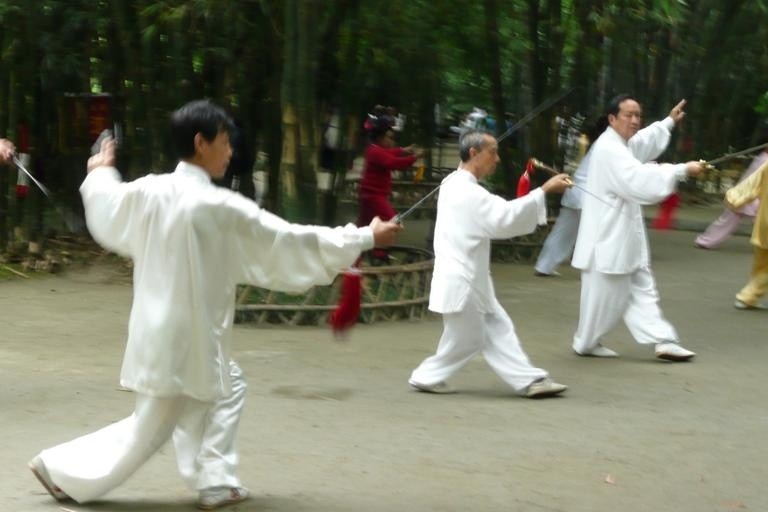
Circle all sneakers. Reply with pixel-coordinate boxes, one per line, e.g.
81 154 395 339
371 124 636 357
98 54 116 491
735 299 768 311
526 376 570 398
654 341 697 361
408 377 460 395
587 342 619 359
195 486 249 510
29 452 69 503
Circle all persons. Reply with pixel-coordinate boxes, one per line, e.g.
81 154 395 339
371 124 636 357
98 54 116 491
693 148 768 249
450 104 608 183
0 137 19 170
29 98 405 512
408 132 572 398
317 98 428 265
724 163 768 309
572 92 705 361
534 116 615 277
